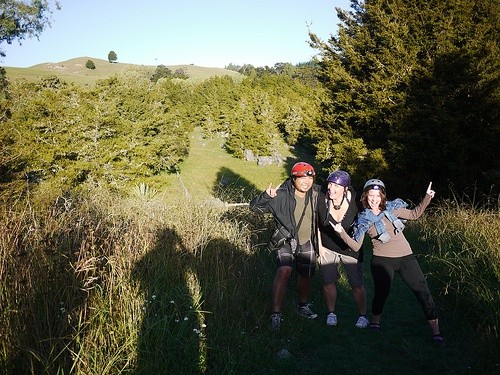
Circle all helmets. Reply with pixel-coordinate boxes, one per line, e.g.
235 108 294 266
326 171 351 187
290 162 315 177
363 178 386 194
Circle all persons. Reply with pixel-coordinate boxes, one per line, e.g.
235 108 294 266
250 162 326 330
329 180 445 345
317 170 369 329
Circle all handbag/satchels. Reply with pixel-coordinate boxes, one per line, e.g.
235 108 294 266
266 224 293 255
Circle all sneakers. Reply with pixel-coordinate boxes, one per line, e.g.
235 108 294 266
355 313 369 329
326 312 338 326
369 322 380 333
296 302 318 320
432 334 444 345
270 311 283 332
